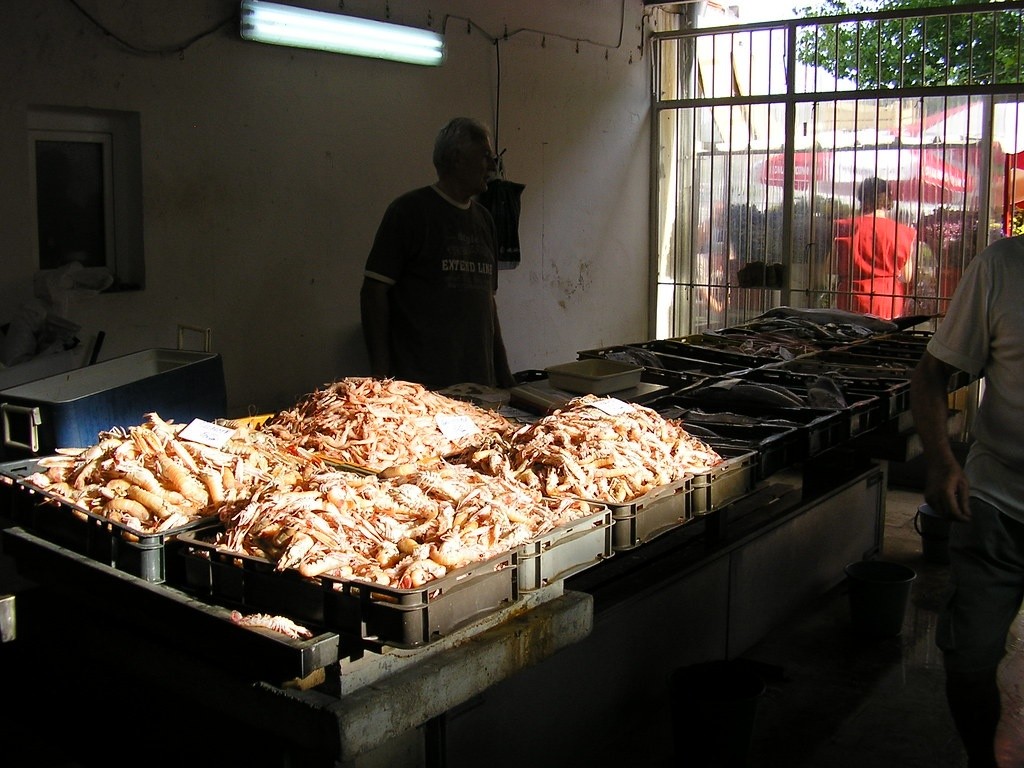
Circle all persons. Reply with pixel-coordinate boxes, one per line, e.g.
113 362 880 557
825 178 919 323
909 229 1023 768
357 115 514 395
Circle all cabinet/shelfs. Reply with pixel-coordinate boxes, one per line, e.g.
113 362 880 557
428 456 890 768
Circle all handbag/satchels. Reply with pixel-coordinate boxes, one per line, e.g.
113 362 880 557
487 154 527 262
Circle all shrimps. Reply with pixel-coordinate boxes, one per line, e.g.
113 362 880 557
229 609 314 643
1 374 725 603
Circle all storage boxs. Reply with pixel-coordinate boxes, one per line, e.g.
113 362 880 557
0 323 985 651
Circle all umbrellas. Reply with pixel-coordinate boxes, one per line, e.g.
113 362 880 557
755 127 973 206
908 99 1024 216
